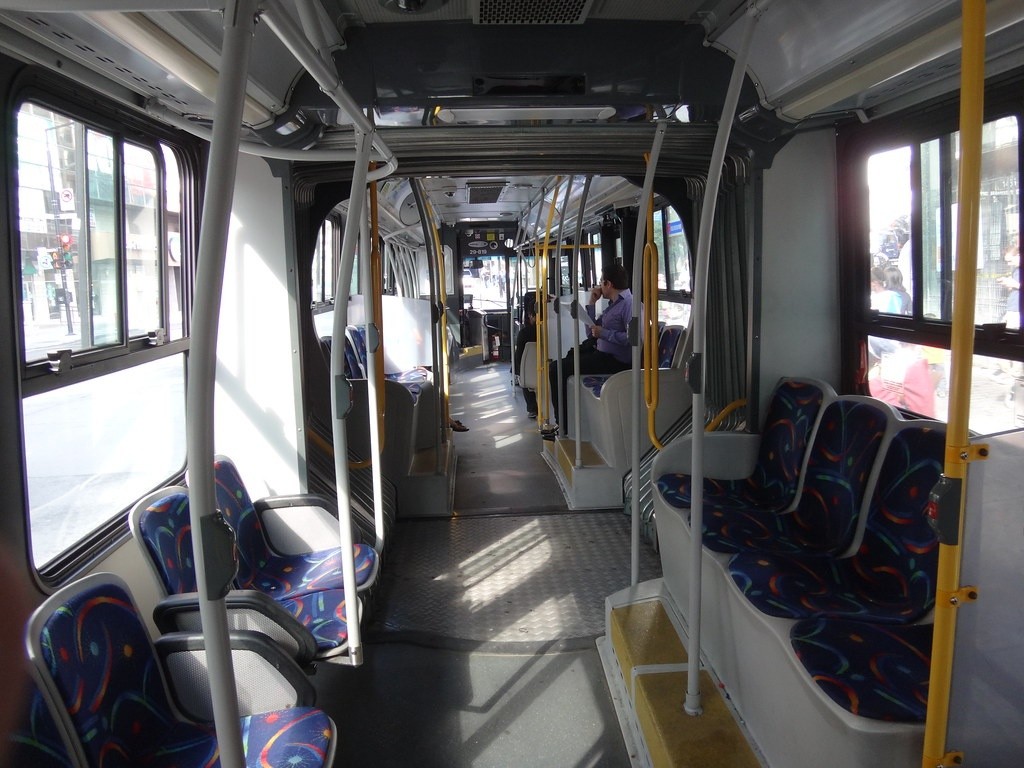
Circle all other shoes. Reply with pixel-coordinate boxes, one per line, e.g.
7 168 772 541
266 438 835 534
511 378 519 386
528 411 537 418
451 420 470 432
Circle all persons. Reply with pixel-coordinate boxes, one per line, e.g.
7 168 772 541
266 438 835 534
430 300 469 432
540 263 645 441
482 270 511 299
510 302 542 418
870 235 1023 418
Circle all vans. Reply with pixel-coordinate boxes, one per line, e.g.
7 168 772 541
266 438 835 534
463 270 473 286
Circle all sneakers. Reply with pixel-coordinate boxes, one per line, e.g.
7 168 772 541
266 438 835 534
540 425 567 441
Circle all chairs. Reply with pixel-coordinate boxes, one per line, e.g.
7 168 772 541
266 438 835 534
515 342 537 389
23 572 337 768
566 322 683 465
127 453 380 660
650 376 977 768
320 322 428 406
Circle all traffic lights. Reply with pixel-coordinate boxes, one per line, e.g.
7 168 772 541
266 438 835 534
62 234 74 270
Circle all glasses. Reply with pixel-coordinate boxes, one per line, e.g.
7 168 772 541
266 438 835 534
599 280 607 284
